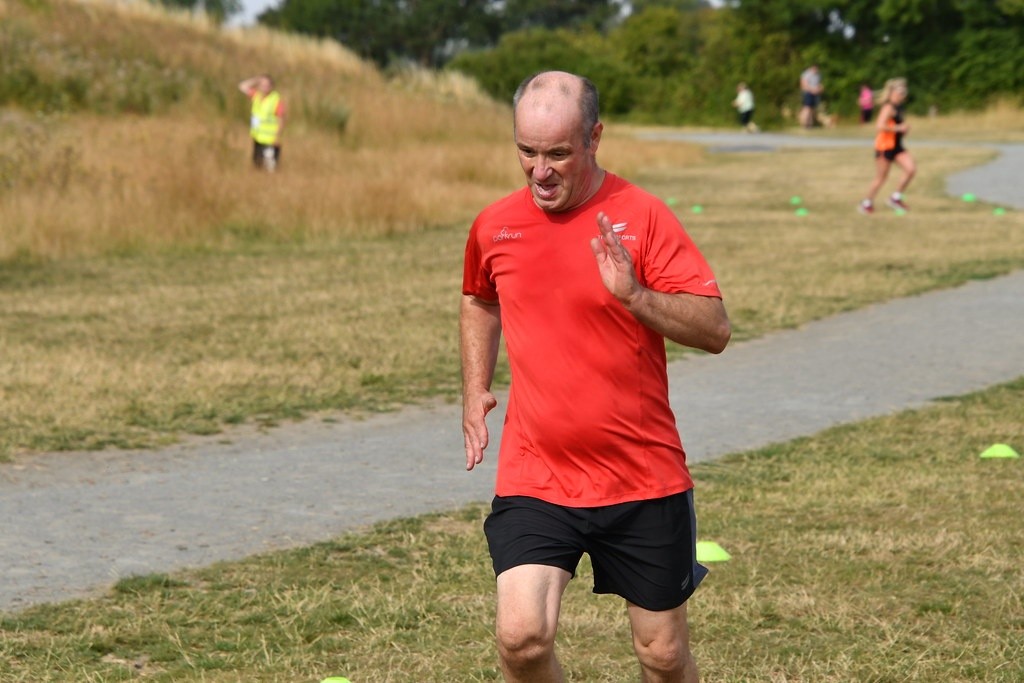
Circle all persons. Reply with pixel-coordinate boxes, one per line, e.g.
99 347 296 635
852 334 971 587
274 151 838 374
237 72 287 172
860 77 915 215
799 63 877 131
730 81 762 134
460 70 731 683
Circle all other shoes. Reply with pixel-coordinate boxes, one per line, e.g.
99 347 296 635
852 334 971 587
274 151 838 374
857 203 874 213
887 194 910 211
